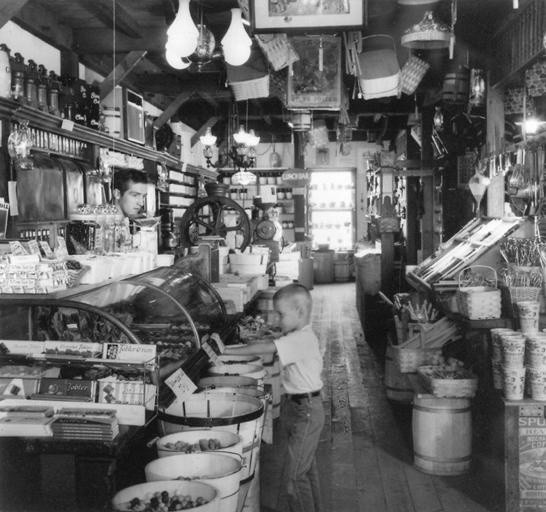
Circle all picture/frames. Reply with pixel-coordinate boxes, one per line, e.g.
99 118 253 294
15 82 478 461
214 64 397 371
248 1 368 32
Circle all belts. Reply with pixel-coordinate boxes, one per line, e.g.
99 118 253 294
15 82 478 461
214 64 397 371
285 390 319 405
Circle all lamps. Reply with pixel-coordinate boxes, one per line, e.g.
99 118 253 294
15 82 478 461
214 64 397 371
163 0 253 71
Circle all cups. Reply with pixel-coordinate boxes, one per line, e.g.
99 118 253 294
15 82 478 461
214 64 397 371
490 327 546 400
519 300 542 335
309 181 355 251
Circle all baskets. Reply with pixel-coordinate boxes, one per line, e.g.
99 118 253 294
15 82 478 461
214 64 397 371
417 362 480 400
455 264 502 321
393 320 445 372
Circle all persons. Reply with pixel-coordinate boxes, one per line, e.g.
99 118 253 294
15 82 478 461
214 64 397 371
210 284 324 512
108 168 150 221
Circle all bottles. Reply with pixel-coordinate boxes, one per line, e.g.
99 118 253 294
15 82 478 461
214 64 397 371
49 71 58 113
12 52 24 99
70 204 126 256
37 65 48 110
139 226 158 269
58 76 100 131
17 228 49 248
25 59 37 106
0 45 11 97
